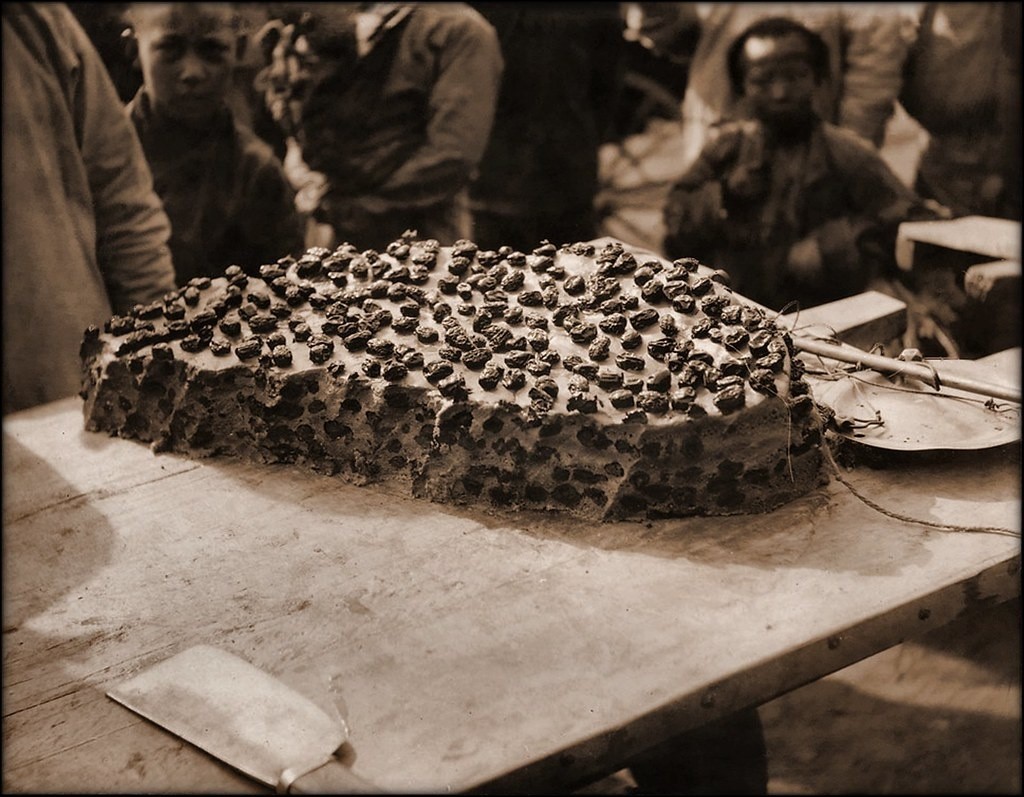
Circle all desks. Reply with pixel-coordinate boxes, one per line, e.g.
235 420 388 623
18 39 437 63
0 285 1024 797
895 214 1024 304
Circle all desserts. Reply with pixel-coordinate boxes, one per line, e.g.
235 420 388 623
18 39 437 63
78 228 838 527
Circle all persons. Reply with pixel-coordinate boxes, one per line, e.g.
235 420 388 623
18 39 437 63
680 2 1022 224
654 17 919 313
119 2 307 279
3 2 175 414
279 2 505 248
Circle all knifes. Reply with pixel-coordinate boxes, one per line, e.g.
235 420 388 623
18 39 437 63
104 643 382 793
